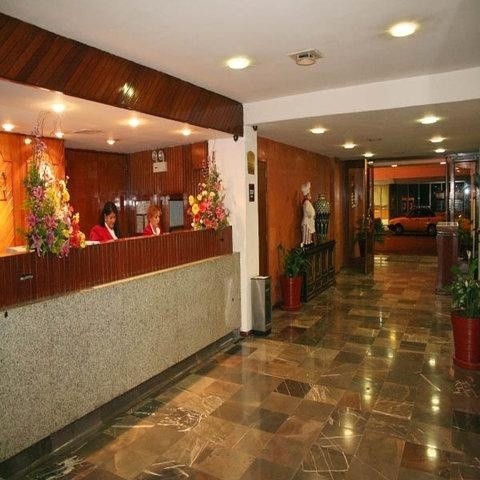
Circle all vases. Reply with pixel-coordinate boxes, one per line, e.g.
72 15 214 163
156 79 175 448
313 194 331 242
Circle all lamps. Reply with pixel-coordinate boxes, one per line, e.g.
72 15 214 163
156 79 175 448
151 150 168 173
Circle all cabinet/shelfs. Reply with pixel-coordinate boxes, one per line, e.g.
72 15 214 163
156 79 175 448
302 240 337 302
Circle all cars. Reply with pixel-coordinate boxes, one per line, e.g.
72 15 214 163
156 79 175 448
388 205 472 237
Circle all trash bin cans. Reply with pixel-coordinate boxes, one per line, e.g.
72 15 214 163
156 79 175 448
251 276 271 337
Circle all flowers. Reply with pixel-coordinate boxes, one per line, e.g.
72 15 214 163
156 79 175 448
185 146 229 231
16 112 87 257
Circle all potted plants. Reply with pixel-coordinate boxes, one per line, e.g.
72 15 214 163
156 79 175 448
448 250 480 370
278 243 303 311
354 215 384 256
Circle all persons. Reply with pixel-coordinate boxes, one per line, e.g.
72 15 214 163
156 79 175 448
302 191 318 245
144 203 163 236
89 202 120 241
